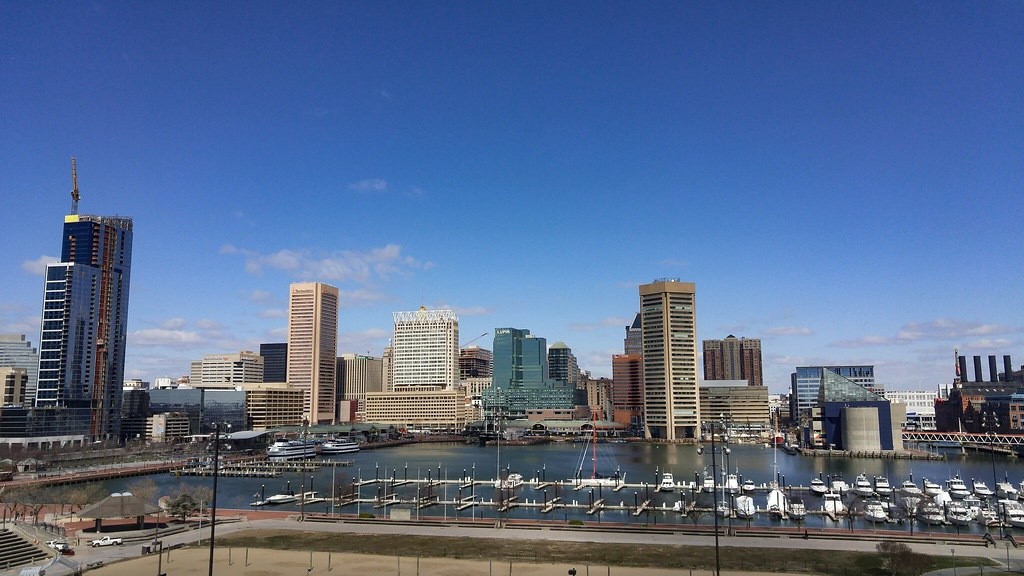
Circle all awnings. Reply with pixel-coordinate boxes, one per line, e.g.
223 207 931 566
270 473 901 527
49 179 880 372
218 429 281 440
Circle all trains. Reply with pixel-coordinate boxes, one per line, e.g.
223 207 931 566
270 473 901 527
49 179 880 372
466 421 625 429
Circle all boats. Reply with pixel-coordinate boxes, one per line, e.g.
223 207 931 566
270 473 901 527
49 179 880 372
266 493 297 503
672 500 687 513
899 482 922 496
810 478 827 494
904 496 921 520
726 472 739 494
566 404 625 487
780 431 799 455
880 498 905 523
1018 480 1024 500
853 474 873 497
914 499 942 526
996 471 1020 501
790 502 804 518
736 495 756 519
977 498 1002 526
696 419 733 575
659 472 676 491
924 480 945 497
943 501 972 525
744 479 755 494
962 494 980 520
768 429 785 447
861 498 887 523
321 429 360 456
973 482 995 500
494 472 524 490
982 410 1001 542
702 475 714 493
830 473 848 495
268 433 317 464
997 497 1024 529
932 493 952 517
946 474 969 499
824 488 844 521
764 489 790 523
874 475 891 496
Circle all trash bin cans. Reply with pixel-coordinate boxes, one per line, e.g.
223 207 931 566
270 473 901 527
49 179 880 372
142 545 151 554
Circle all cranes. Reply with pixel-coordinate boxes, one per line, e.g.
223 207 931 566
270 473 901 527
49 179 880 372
460 332 489 349
67 153 83 215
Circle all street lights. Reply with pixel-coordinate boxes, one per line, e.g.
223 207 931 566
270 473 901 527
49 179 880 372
1005 543 1014 572
979 564 984 576
298 415 311 522
950 548 957 576
489 385 508 491
202 418 235 576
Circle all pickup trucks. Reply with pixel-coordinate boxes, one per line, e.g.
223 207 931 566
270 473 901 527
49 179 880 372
92 535 124 547
45 541 70 553
